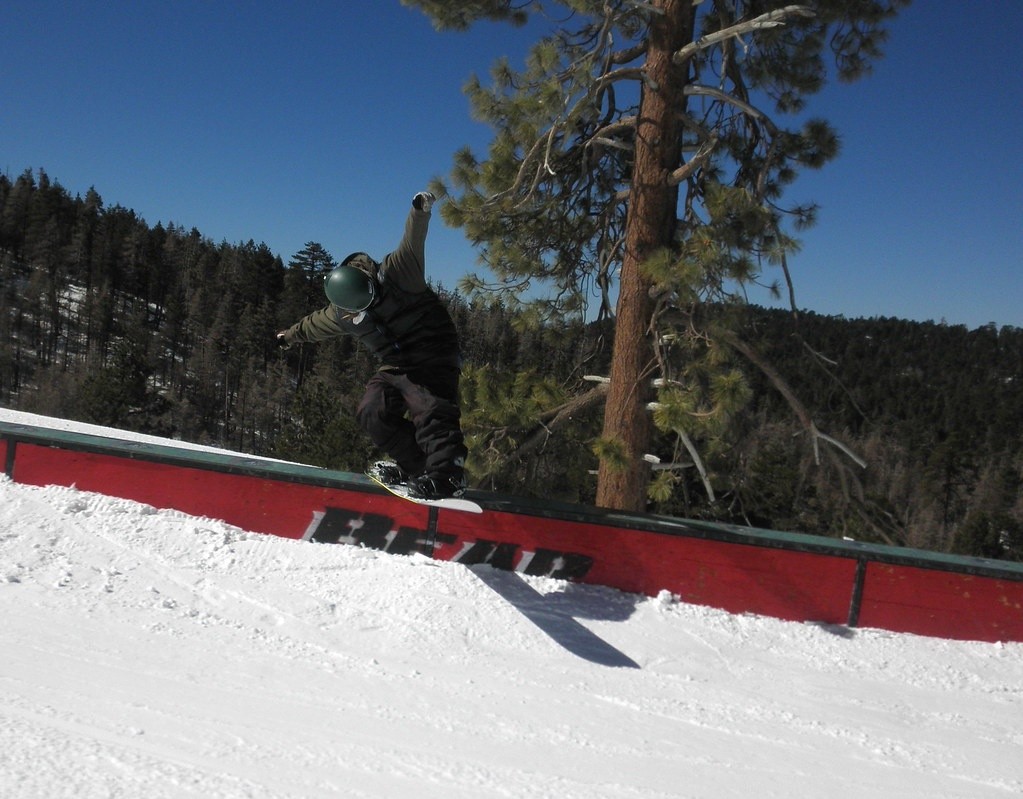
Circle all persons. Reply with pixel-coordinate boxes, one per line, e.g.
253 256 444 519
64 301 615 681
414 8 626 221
276 191 469 501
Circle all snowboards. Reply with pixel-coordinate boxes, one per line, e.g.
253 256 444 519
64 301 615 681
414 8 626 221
363 468 485 515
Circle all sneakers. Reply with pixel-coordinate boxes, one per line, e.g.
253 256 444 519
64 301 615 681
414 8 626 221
406 471 468 499
368 461 426 484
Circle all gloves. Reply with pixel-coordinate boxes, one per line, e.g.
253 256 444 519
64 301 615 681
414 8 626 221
275 329 294 350
413 192 436 214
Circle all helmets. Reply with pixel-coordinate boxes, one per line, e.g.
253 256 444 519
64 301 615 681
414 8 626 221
323 266 373 311
